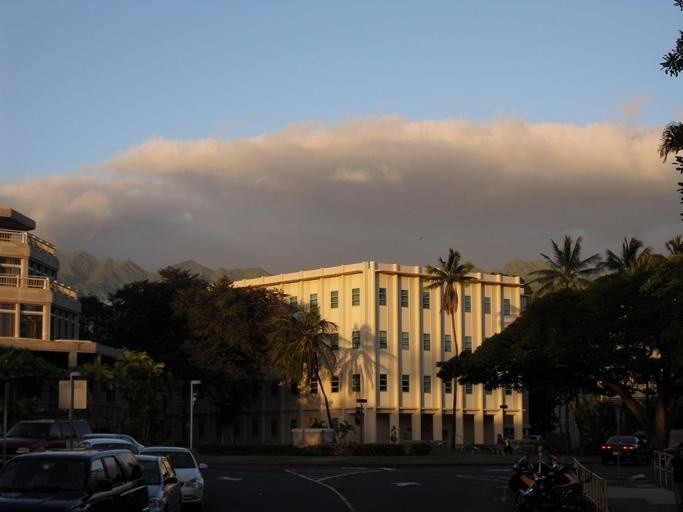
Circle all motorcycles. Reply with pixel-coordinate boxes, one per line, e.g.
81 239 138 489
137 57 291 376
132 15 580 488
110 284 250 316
486 443 506 455
508 444 584 510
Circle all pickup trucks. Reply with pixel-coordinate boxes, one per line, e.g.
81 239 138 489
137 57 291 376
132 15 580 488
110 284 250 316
510 435 541 450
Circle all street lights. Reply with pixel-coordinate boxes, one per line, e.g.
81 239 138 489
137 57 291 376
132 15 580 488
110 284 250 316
67 369 81 419
354 398 368 444
189 378 203 456
498 404 508 452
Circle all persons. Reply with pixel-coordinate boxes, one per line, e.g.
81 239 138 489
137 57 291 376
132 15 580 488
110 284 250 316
666 442 683 505
496 434 505 456
306 418 320 428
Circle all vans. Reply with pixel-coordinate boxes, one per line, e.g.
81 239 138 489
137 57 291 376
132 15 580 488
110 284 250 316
82 434 145 452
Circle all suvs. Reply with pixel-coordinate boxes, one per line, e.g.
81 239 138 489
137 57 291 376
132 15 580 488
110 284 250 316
0 449 149 512
0 418 91 454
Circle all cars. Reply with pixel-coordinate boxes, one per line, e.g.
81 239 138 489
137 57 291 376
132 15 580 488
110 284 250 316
659 442 682 465
130 454 184 511
138 447 207 506
599 436 645 462
78 440 139 454
